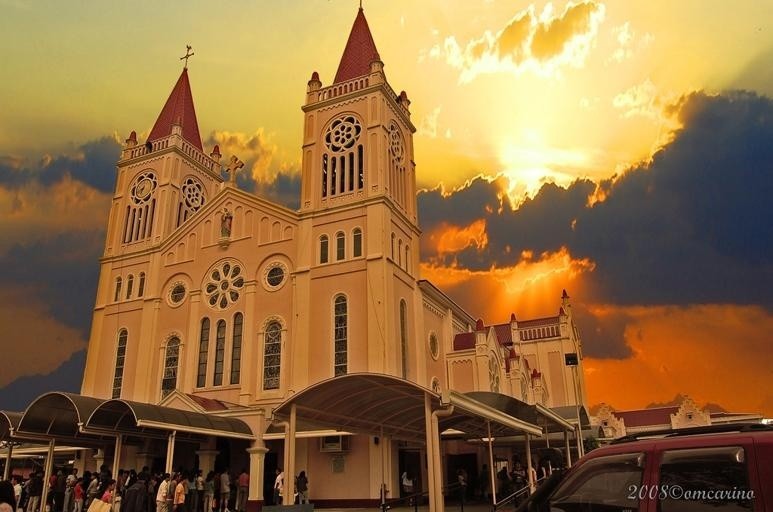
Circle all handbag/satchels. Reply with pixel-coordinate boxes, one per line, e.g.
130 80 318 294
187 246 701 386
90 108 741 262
87 496 112 512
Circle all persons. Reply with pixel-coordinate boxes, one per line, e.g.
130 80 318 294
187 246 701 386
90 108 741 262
0 464 250 512
458 470 468 491
497 458 552 497
297 471 311 504
220 208 232 237
480 462 490 503
273 468 284 504
401 464 417 494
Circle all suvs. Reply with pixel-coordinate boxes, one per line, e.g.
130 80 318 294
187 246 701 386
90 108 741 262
522 420 772 510
